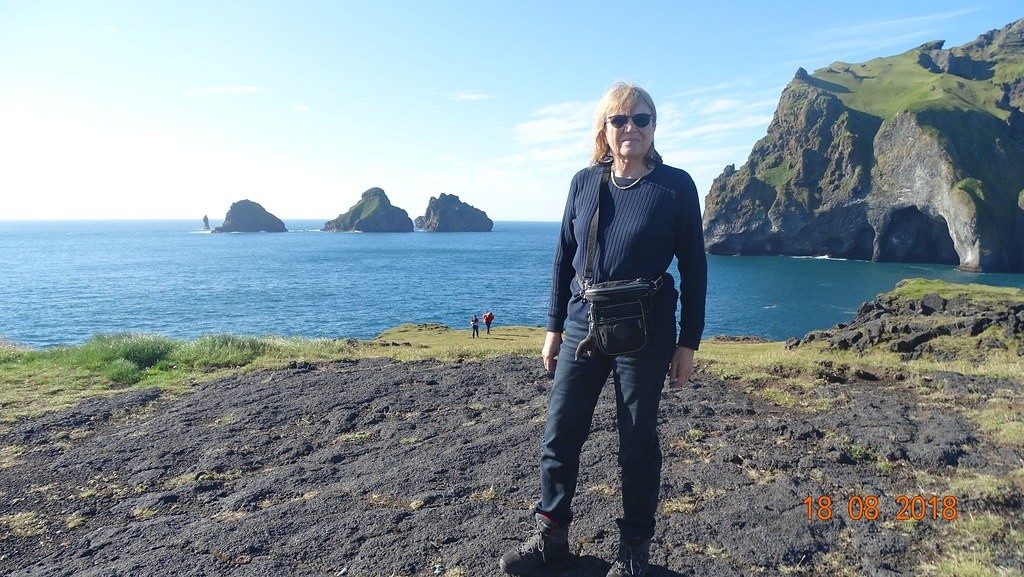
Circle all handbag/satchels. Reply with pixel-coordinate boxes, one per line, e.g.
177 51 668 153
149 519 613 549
572 278 652 364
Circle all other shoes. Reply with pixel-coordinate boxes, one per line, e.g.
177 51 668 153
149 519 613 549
487 332 490 334
472 336 475 338
476 336 478 338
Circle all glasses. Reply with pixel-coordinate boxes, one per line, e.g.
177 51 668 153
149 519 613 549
604 114 653 129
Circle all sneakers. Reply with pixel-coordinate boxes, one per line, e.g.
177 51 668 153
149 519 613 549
605 533 650 577
499 513 570 575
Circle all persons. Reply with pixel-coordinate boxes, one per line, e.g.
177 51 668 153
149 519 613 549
470 314 479 338
483 312 495 334
498 80 709 576
